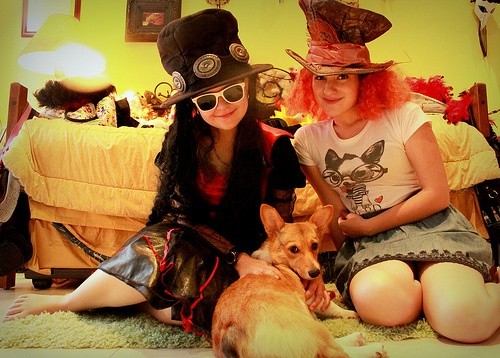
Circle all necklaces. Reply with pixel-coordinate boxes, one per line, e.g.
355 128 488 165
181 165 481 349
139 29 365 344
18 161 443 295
213 146 232 168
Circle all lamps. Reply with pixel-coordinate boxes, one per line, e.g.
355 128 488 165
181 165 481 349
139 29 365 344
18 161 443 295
16 13 105 79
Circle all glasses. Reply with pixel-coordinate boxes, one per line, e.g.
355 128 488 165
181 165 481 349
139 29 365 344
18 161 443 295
191 77 248 113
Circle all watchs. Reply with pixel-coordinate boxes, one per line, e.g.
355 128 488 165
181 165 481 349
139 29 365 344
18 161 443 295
224 244 243 266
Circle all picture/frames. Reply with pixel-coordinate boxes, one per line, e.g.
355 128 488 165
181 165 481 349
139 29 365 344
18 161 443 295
124 0 181 41
21 0 81 38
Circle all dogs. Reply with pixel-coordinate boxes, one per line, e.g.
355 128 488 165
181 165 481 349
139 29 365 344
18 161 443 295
211 203 389 358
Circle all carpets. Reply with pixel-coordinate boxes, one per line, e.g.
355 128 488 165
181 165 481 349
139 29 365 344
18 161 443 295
0 295 442 349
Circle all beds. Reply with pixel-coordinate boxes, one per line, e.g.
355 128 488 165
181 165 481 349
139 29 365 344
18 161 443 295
7 83 490 290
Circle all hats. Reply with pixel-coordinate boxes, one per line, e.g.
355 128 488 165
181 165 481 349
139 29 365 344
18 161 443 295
285 0 394 75
156 9 273 108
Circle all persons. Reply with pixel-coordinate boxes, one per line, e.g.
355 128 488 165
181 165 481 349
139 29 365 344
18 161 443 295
5 7 306 322
286 0 500 345
